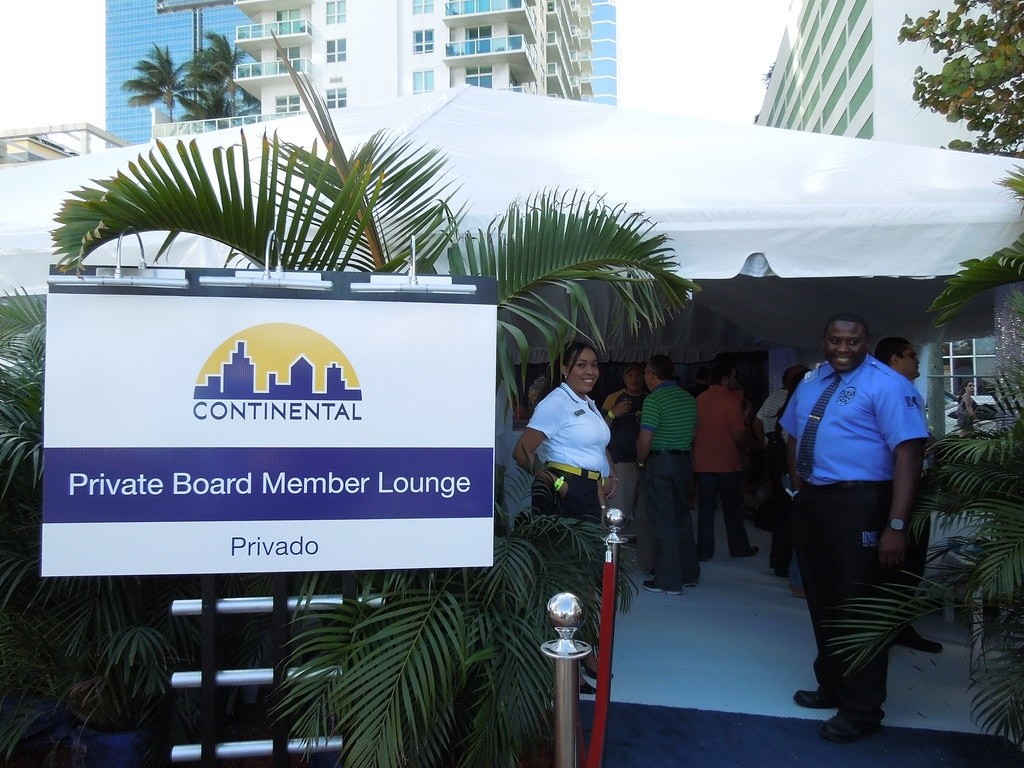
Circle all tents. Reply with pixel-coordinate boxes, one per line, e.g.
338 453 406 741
0 85 1024 299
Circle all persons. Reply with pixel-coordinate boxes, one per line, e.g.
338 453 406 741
874 335 944 653
512 339 619 525
601 362 650 545
634 355 701 596
752 364 815 576
955 380 978 437
777 312 930 742
687 359 759 560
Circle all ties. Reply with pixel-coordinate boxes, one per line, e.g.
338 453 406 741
796 374 844 479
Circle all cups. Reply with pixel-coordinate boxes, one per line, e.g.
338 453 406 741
621 397 632 405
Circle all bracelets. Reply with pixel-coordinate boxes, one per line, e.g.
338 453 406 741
636 457 645 467
608 410 616 420
608 475 619 482
553 475 565 491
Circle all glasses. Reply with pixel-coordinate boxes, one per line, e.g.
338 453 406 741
899 352 918 359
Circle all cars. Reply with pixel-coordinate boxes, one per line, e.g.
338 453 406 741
945 398 1007 438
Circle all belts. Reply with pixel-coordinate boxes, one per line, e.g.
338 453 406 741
649 449 691 454
547 461 600 480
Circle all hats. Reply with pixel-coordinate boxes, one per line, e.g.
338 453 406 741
624 361 643 374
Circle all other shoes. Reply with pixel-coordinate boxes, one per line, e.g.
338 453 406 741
643 580 680 595
684 578 697 586
775 568 791 578
892 624 943 652
732 545 759 557
790 584 806 598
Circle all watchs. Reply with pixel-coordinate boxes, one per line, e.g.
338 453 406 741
886 518 906 531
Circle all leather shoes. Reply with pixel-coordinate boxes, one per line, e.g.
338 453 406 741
818 712 880 741
794 688 837 709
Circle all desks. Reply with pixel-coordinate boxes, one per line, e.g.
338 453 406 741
944 545 988 656
